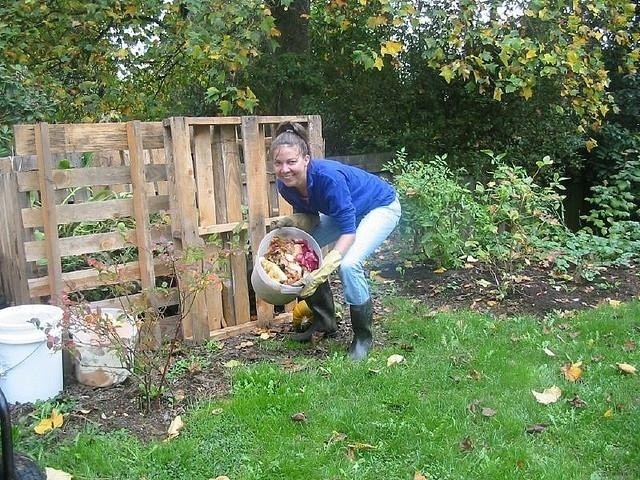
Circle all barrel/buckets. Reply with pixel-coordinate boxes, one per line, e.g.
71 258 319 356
0 302 64 405
66 307 138 388
250 225 323 307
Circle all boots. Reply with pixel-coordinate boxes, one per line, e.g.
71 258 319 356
293 279 337 342
349 298 372 359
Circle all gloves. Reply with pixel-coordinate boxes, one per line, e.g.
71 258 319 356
275 213 319 230
292 250 342 298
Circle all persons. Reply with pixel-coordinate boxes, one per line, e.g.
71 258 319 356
266 119 403 364
